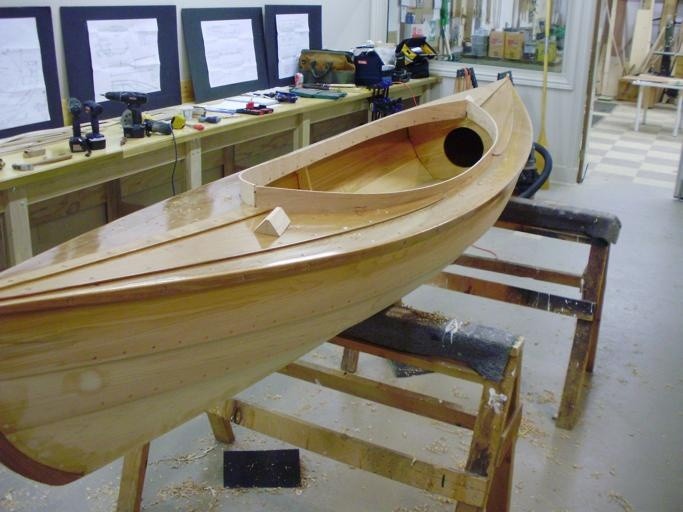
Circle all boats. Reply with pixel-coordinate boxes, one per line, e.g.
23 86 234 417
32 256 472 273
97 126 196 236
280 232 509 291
1 81 532 487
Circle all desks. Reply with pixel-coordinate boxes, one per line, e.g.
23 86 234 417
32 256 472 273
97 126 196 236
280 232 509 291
1 69 439 266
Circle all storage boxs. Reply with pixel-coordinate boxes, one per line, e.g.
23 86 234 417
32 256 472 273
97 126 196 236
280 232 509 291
488 29 526 63
